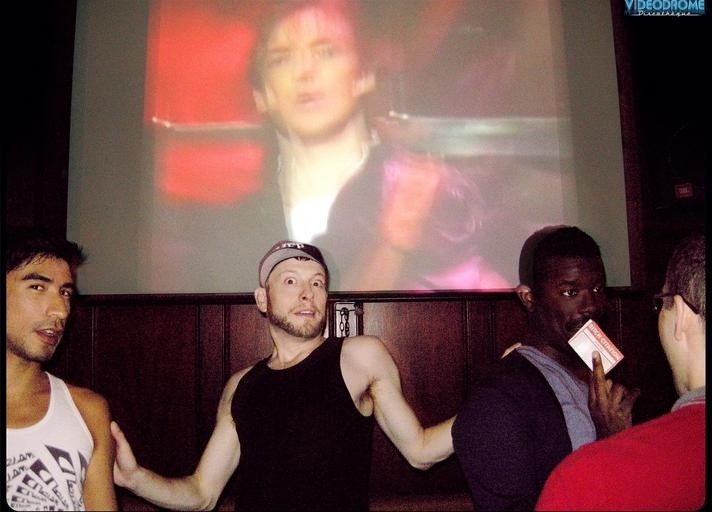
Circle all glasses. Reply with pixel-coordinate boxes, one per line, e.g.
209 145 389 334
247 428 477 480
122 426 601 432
652 292 699 315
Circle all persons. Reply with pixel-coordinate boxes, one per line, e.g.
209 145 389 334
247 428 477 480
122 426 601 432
214 6 512 293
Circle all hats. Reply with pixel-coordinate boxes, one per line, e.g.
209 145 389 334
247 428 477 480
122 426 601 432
258 240 330 288
